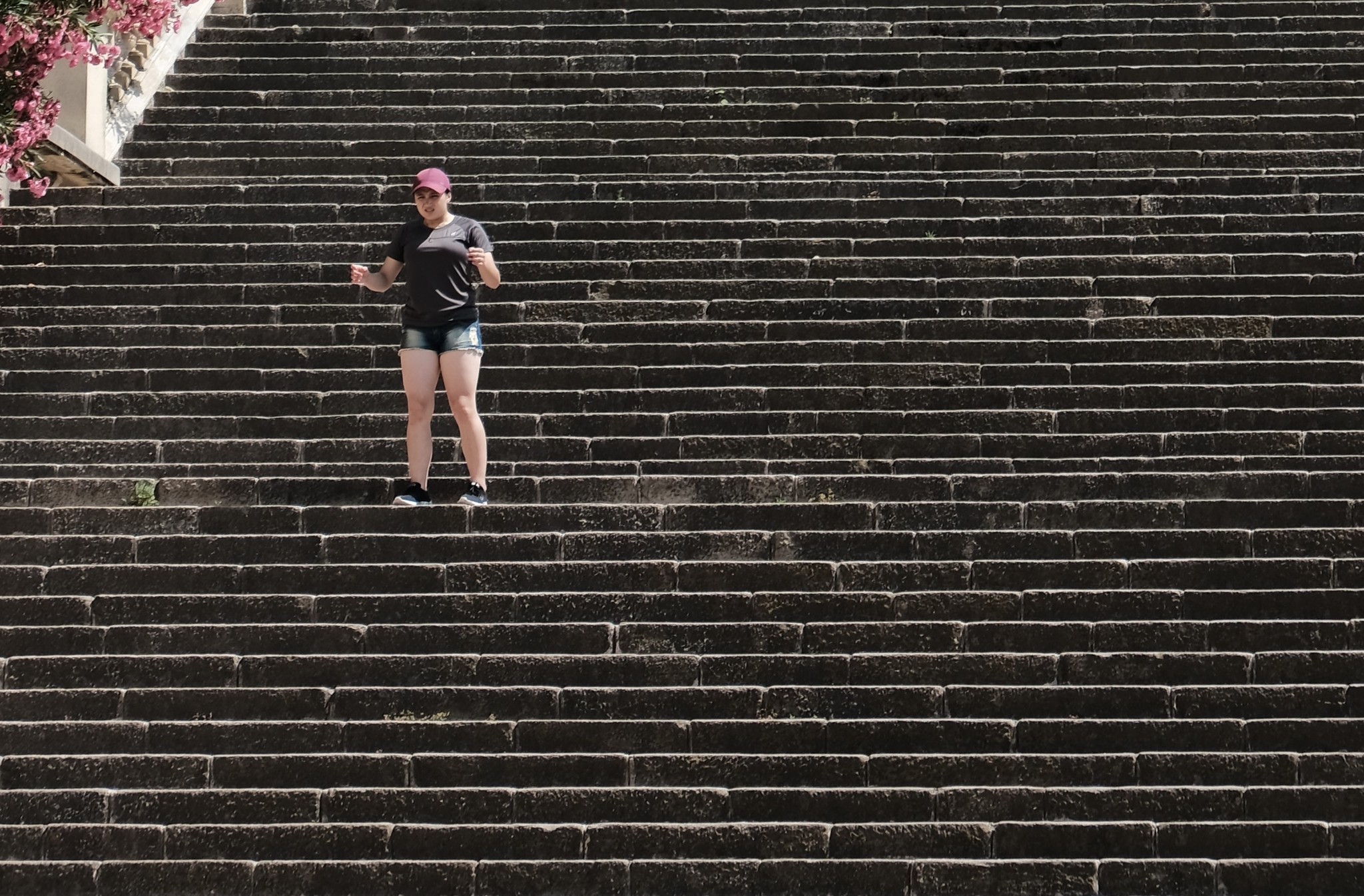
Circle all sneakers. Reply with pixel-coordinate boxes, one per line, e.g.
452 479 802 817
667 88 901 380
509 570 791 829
457 482 488 506
393 481 432 505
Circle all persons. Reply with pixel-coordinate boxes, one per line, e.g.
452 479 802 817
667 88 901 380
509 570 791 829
349 167 500 508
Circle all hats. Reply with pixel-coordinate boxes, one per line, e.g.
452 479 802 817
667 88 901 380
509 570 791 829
412 168 451 194
